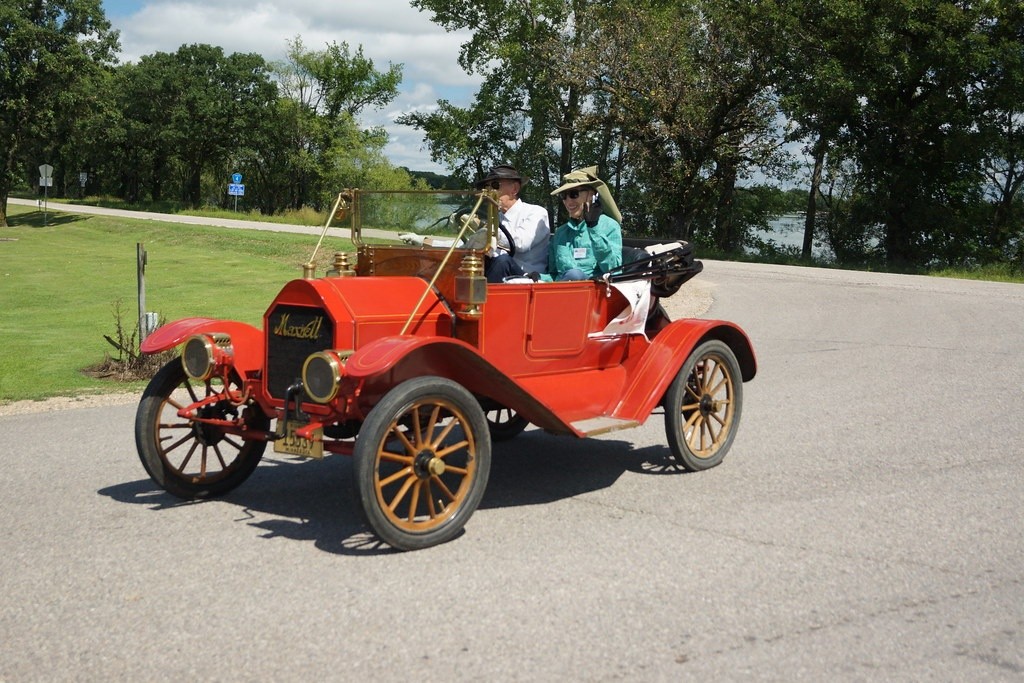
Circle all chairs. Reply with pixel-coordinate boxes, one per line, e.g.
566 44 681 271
620 243 650 277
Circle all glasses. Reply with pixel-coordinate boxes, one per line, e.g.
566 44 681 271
484 181 513 190
561 188 589 200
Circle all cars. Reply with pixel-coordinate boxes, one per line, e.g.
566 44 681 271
130 172 761 554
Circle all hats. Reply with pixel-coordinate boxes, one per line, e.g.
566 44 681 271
473 165 530 191
550 166 623 225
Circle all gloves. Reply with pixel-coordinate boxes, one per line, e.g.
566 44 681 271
448 213 475 229
525 271 541 283
582 199 604 227
397 231 430 247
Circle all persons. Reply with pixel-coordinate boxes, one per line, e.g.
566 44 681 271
526 168 624 284
446 163 552 282
